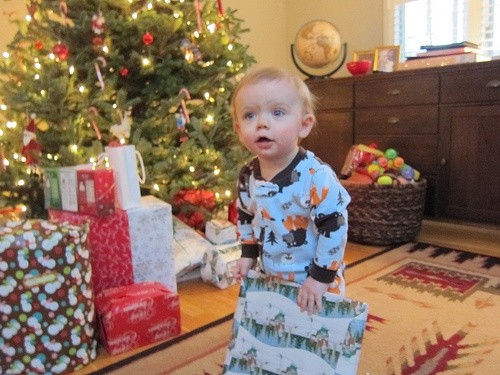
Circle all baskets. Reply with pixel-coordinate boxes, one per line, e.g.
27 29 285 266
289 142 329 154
338 174 426 245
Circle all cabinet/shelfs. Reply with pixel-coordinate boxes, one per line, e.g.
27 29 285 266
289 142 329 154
300 60 500 227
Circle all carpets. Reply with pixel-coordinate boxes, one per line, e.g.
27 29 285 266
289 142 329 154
85 240 500 375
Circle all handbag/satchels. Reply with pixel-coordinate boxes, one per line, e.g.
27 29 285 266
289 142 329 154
92 144 146 210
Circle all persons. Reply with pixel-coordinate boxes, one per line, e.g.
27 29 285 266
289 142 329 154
230 66 351 316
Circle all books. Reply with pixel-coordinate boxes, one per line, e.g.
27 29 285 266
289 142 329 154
405 41 491 70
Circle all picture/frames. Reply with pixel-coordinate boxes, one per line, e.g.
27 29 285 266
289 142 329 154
353 45 400 72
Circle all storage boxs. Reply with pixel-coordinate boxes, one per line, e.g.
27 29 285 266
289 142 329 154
0 144 241 375
335 179 428 245
